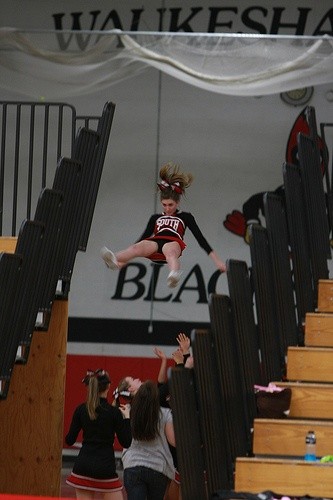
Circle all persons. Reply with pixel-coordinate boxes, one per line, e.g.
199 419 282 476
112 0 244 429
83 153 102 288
116 376 169 474
153 329 193 386
66 368 133 500
102 167 226 288
123 383 177 500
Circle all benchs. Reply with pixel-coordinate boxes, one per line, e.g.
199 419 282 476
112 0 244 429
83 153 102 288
234 279 333 499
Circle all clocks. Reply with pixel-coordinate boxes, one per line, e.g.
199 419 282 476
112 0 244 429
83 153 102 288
280 86 313 107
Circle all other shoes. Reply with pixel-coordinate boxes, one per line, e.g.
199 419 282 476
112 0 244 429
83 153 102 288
102 246 120 271
167 273 178 288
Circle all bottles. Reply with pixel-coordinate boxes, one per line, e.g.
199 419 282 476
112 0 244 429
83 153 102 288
304 430 316 461
